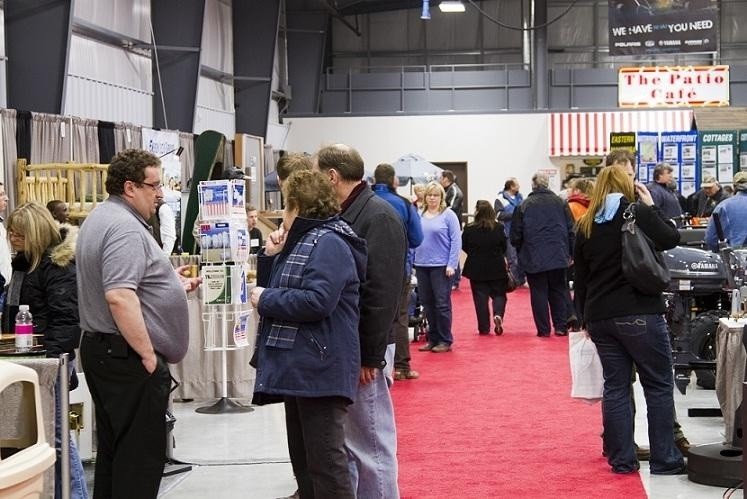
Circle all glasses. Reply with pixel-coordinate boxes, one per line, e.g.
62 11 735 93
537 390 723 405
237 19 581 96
132 180 164 190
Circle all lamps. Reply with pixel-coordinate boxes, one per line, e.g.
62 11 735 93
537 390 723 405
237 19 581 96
440 2 465 13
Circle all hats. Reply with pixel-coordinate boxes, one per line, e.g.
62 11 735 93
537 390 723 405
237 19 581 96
700 175 715 187
734 172 747 183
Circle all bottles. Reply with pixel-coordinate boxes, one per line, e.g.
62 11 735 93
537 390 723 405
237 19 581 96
14 304 34 353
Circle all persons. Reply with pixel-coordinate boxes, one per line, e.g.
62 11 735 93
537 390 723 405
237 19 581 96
495 147 747 477
0 181 12 316
5 201 88 499
74 146 189 499
146 197 183 256
461 199 508 337
48 200 71 224
245 144 462 498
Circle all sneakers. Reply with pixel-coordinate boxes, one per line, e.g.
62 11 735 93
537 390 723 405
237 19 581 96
602 437 691 474
394 343 451 380
480 315 577 337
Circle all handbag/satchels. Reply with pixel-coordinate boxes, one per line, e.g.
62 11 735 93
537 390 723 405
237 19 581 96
621 202 671 295
505 269 516 292
